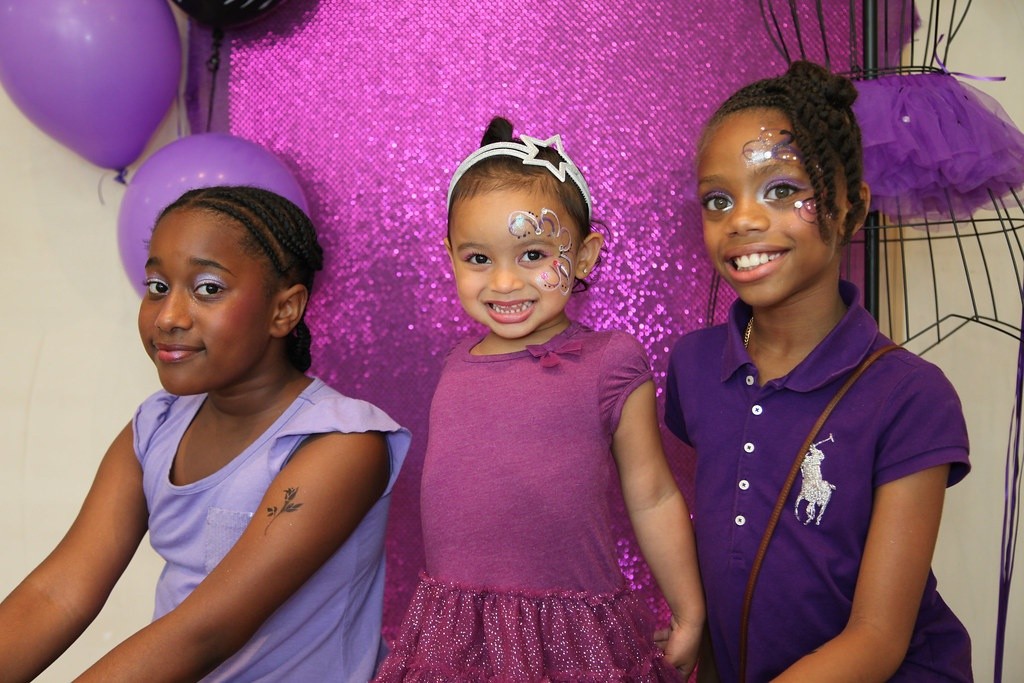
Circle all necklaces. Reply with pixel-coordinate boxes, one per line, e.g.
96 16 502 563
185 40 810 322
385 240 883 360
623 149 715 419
741 314 755 352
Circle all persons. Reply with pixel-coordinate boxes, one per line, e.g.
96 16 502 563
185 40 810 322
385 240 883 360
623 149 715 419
371 116 709 683
0 183 414 683
662 55 977 683
